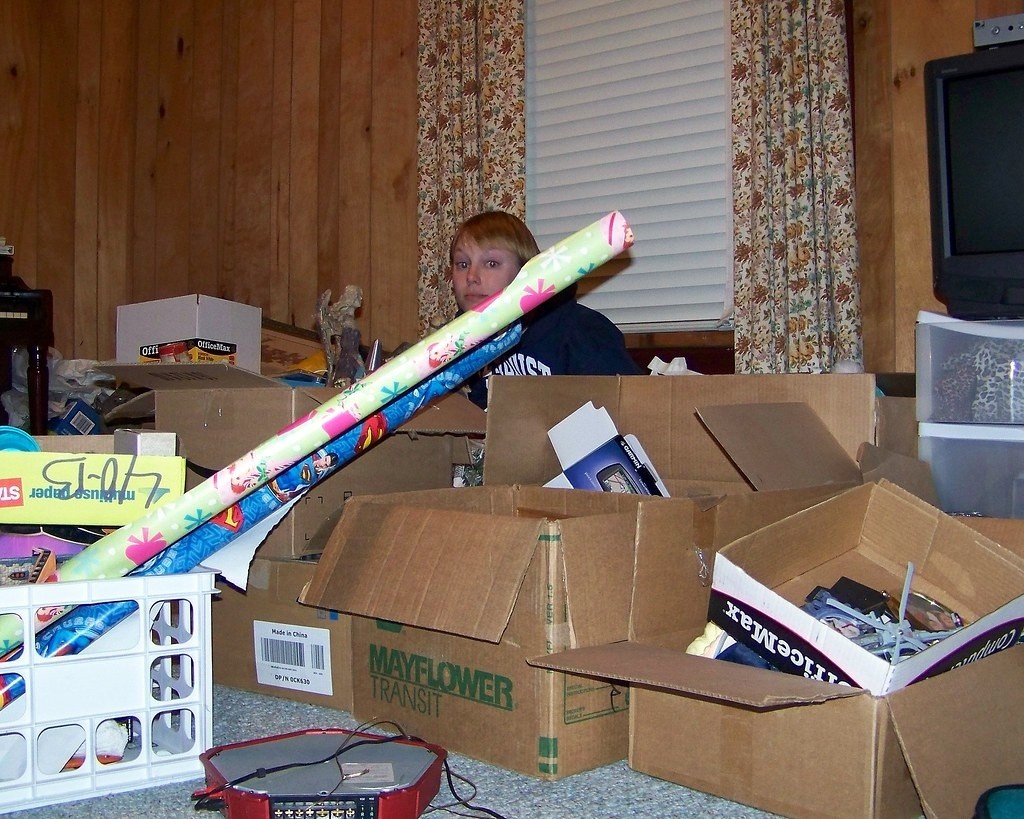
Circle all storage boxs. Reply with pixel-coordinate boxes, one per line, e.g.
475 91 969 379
0 293 1024 817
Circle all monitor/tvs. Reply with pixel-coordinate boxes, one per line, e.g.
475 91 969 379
925 42 1024 320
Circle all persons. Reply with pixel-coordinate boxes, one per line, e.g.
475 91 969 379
448 210 640 413
